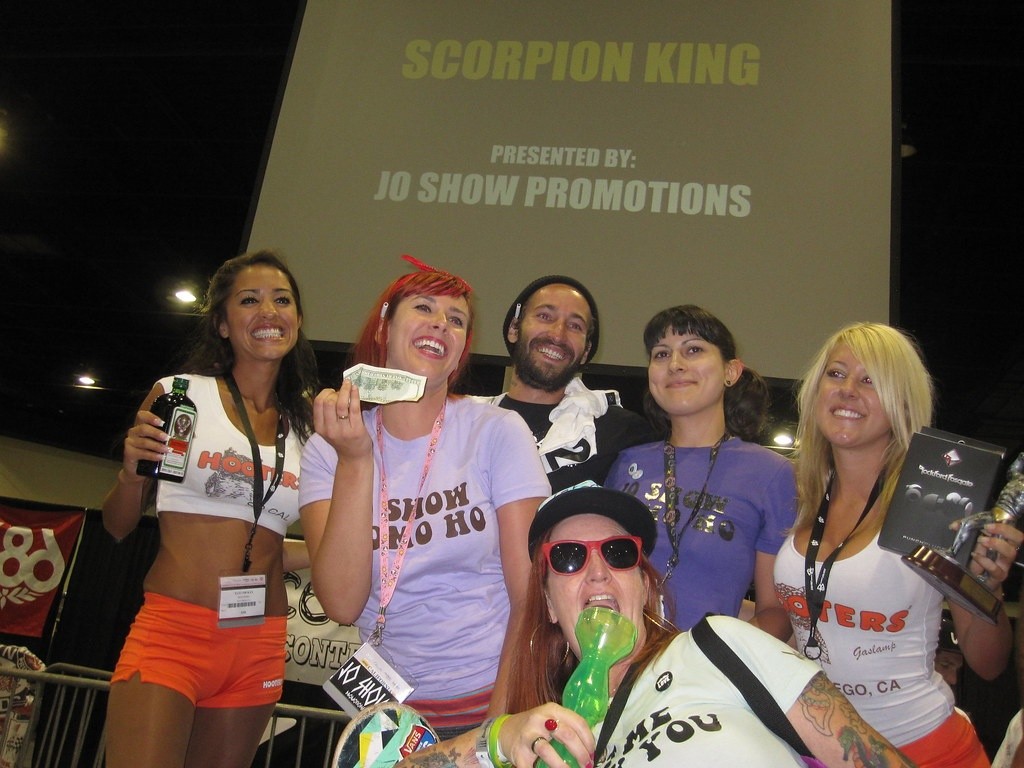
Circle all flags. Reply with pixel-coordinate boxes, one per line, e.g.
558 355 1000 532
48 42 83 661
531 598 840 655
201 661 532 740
0 507 90 641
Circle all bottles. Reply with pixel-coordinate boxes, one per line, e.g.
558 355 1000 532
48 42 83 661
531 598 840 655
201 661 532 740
135 377 198 483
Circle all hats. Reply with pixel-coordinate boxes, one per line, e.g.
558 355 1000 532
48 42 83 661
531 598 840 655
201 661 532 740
503 274 600 368
934 609 962 654
527 479 658 564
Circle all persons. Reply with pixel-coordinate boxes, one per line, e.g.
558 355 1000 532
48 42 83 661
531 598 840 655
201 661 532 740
391 480 925 768
466 275 661 492
603 304 805 647
930 618 963 690
298 271 552 745
102 250 326 767
772 322 1023 768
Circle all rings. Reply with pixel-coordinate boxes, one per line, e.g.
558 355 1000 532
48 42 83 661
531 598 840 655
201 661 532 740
531 737 547 755
338 415 349 419
544 718 560 739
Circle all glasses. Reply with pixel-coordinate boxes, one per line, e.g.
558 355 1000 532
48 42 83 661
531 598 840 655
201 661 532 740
541 534 643 576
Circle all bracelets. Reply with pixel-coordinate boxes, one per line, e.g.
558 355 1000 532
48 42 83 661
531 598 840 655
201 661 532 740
474 713 512 768
